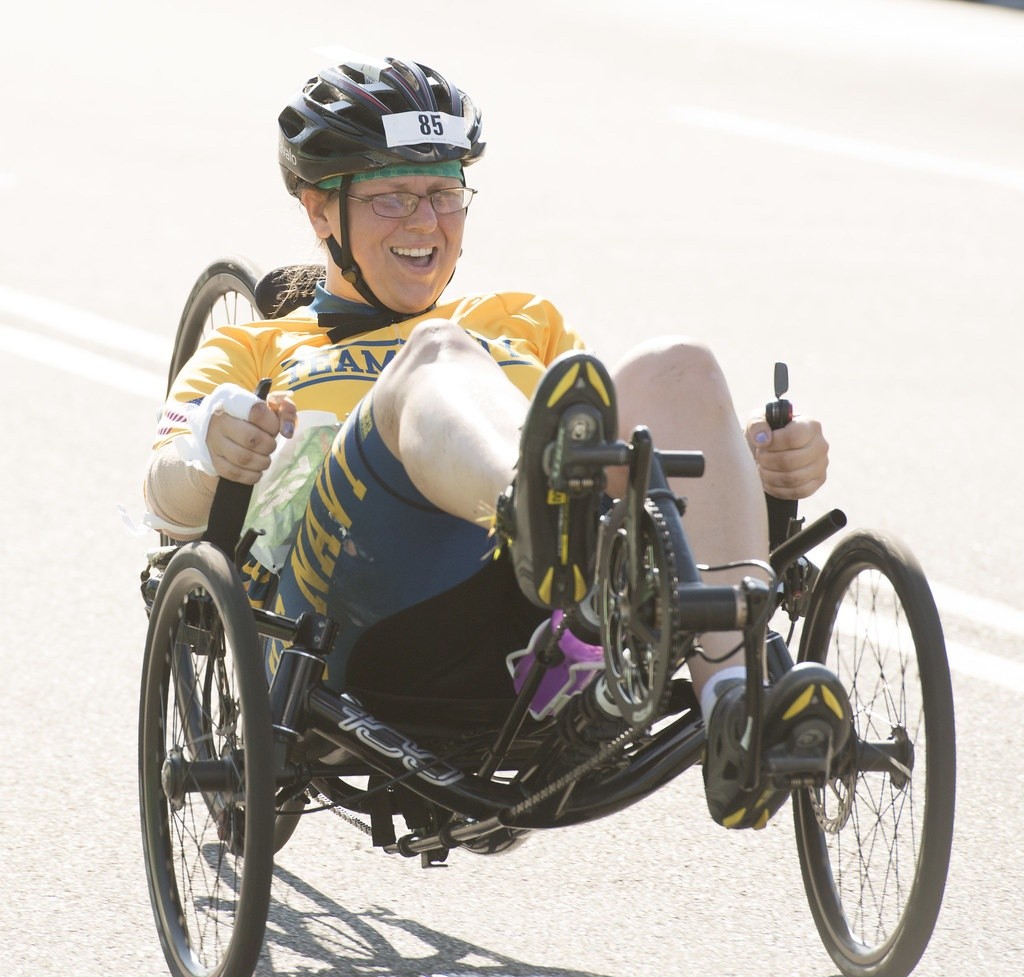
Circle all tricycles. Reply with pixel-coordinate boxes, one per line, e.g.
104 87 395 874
136 257 958 977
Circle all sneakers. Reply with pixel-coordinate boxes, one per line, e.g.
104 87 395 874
489 349 619 610
701 660 854 830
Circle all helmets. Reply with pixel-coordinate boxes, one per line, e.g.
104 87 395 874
277 57 487 197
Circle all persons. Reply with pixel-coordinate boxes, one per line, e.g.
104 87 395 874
145 58 852 829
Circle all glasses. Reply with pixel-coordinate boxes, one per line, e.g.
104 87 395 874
327 187 478 218
